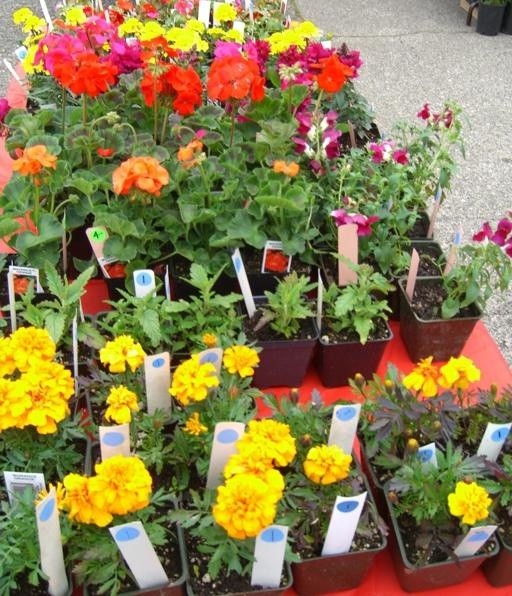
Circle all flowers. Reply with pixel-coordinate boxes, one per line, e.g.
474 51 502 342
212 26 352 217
1 102 473 364
420 214 512 320
1 1 383 154
1 327 511 595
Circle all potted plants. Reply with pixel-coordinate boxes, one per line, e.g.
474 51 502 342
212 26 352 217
242 270 318 389
317 251 394 388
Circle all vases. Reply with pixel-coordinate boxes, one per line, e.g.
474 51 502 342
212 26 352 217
398 277 483 363
